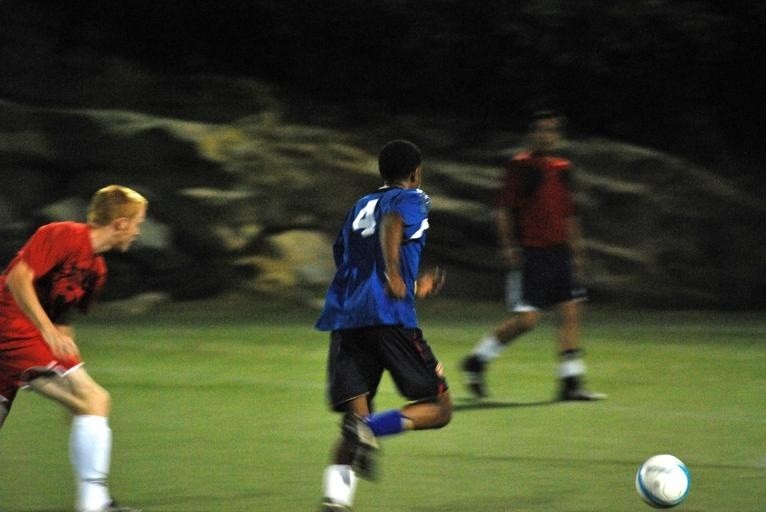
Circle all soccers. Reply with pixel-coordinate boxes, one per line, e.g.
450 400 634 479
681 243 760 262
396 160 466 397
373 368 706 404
635 453 691 508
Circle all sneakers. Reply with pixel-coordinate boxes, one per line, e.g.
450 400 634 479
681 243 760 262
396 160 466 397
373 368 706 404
560 376 608 401
456 353 488 398
323 493 354 510
104 496 143 511
342 414 381 483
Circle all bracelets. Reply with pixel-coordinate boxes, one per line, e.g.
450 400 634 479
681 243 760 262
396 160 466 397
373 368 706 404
383 260 402 279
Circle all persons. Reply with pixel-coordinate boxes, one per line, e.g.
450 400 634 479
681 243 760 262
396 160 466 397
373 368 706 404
313 139 452 512
1 184 149 511
462 109 610 400
